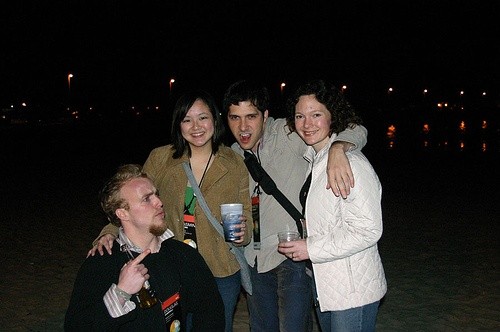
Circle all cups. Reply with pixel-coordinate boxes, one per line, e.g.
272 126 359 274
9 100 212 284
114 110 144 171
220 204 243 242
277 232 299 243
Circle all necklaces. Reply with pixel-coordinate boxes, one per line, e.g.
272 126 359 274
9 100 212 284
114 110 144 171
184 153 212 213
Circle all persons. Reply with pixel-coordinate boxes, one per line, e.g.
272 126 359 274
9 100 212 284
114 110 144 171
86 85 254 332
277 75 388 332
222 78 369 332
65 163 227 332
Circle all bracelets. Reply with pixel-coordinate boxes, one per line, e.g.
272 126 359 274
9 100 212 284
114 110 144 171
114 287 132 301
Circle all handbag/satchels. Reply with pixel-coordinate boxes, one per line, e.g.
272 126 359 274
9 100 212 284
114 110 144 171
230 245 254 296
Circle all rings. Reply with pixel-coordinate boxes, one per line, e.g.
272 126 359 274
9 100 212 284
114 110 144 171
292 252 294 258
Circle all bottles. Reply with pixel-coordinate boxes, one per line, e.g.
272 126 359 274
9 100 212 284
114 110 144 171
121 244 159 311
